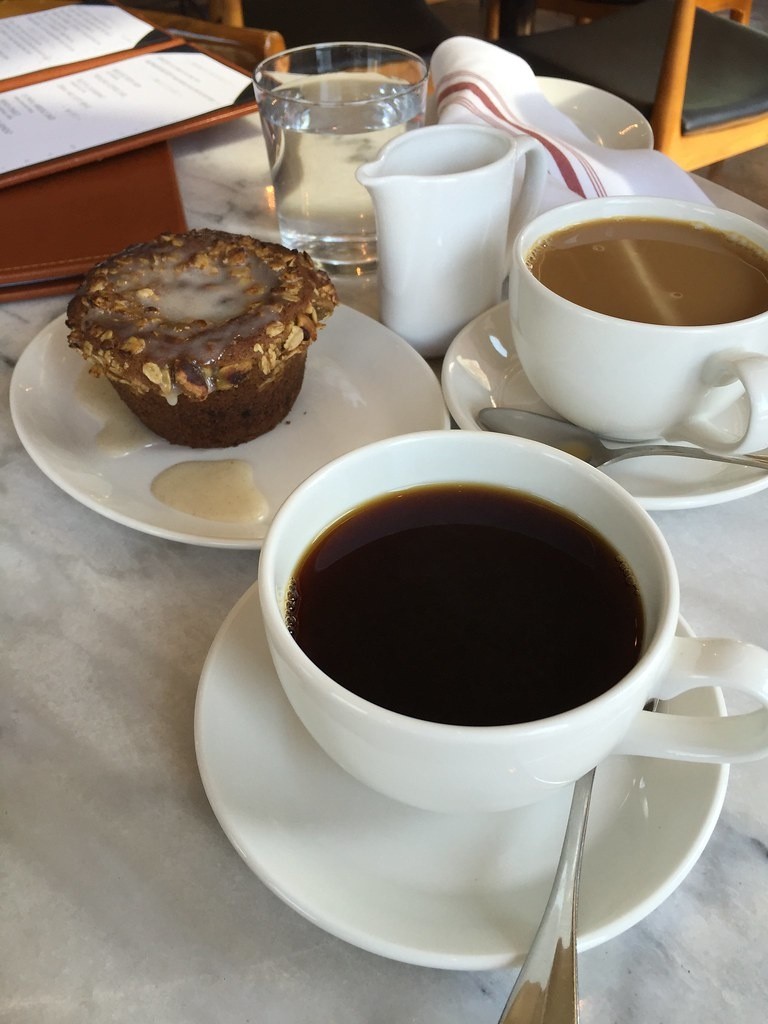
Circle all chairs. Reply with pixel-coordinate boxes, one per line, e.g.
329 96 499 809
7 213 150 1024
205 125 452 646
478 0 768 173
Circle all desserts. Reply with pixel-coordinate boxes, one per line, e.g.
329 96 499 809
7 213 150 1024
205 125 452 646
64 226 340 450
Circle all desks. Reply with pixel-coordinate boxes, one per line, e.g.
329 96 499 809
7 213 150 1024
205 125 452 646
0 68 768 1024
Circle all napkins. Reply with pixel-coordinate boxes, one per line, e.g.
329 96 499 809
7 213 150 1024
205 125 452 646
422 37 712 283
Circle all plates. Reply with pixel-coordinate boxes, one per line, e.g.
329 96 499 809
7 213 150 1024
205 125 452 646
440 300 768 511
9 285 448 550
531 75 654 170
193 580 733 975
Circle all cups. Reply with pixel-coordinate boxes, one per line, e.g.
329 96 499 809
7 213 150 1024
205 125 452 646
254 427 768 817
508 196 768 459
251 40 428 276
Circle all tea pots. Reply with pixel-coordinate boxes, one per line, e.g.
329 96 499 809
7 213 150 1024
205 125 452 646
354 122 551 359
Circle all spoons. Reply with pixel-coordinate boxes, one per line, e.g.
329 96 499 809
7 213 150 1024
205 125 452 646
474 405 768 496
